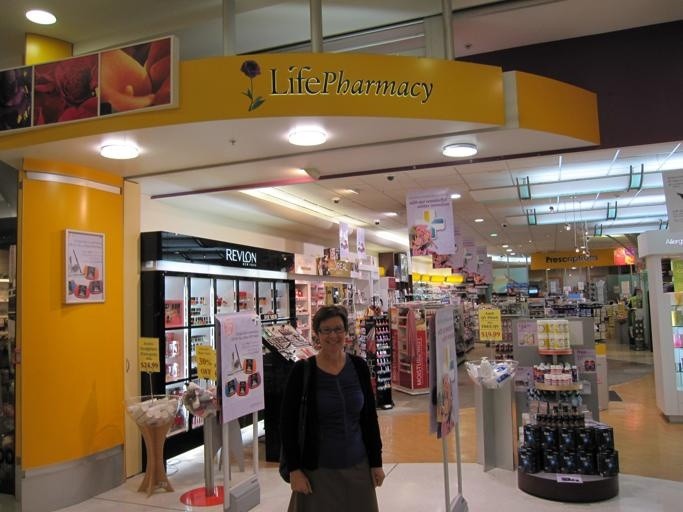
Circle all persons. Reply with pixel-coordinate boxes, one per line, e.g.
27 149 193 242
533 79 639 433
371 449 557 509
474 293 495 348
278 304 386 512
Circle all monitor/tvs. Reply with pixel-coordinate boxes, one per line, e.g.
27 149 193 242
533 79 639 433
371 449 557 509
528 284 539 297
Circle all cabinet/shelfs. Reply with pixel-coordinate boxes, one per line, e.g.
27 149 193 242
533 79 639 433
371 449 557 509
466 285 611 493
0 278 10 318
140 231 467 473
612 233 681 424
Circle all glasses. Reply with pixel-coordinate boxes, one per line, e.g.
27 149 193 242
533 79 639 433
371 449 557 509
317 326 347 334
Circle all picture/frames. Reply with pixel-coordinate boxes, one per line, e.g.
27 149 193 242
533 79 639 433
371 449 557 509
62 228 106 307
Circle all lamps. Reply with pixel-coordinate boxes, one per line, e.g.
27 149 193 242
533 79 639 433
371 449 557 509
97 143 144 162
561 198 591 257
440 143 479 159
289 123 329 148
303 166 322 182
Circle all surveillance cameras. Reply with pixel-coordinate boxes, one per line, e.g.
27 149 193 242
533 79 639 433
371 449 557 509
333 198 339 203
501 223 508 228
374 220 380 225
385 172 396 182
